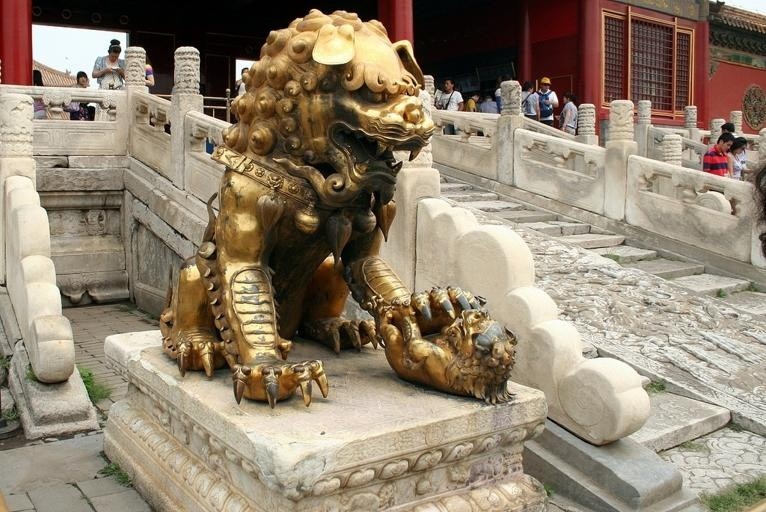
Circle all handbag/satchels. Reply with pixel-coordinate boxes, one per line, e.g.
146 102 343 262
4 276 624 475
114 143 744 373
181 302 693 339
521 93 532 114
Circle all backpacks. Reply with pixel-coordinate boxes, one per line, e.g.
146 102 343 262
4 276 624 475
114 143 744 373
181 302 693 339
536 91 554 118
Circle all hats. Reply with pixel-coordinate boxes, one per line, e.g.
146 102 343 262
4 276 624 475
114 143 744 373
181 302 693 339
540 77 551 84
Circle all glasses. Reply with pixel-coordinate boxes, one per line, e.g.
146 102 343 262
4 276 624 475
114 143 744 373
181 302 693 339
544 83 552 86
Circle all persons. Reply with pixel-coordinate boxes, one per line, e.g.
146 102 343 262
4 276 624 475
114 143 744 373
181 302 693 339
721 123 735 135
728 137 754 180
33 70 47 120
91 39 125 121
70 71 92 120
234 68 249 97
434 76 579 136
145 53 155 87
703 132 735 178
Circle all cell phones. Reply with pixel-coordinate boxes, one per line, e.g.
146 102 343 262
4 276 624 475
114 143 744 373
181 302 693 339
111 66 119 70
